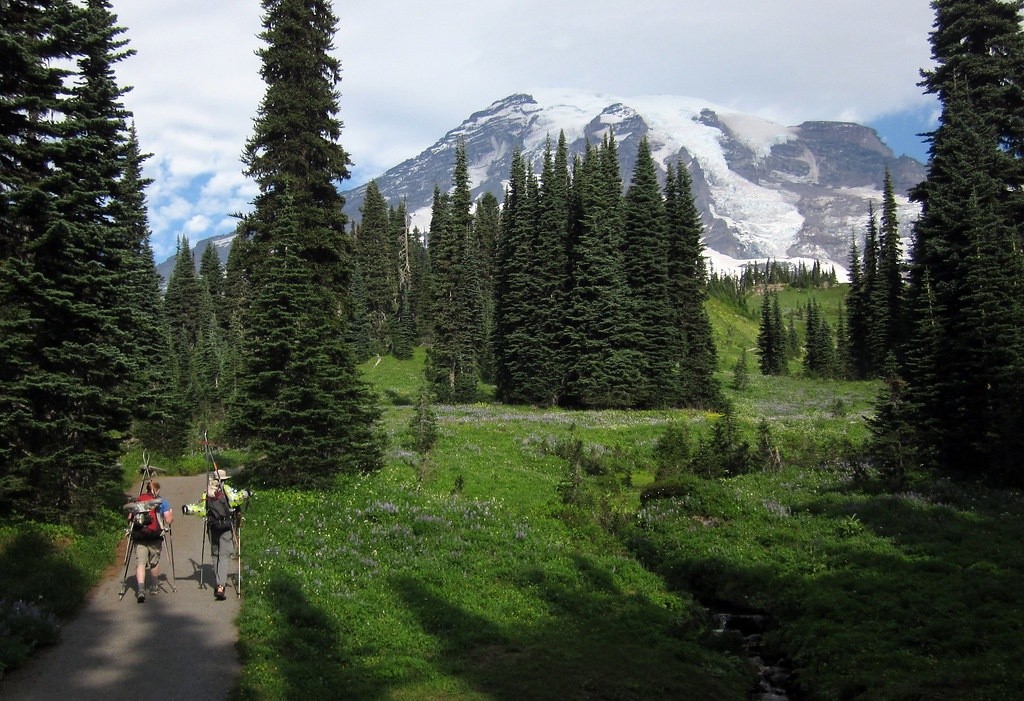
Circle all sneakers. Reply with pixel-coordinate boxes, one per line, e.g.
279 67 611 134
135 584 159 603
214 584 226 600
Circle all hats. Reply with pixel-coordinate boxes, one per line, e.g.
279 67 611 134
212 469 231 481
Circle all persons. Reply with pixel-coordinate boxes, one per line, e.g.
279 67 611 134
182 470 254 599
125 481 174 604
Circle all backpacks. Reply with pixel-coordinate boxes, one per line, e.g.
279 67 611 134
205 490 232 535
131 506 162 540
